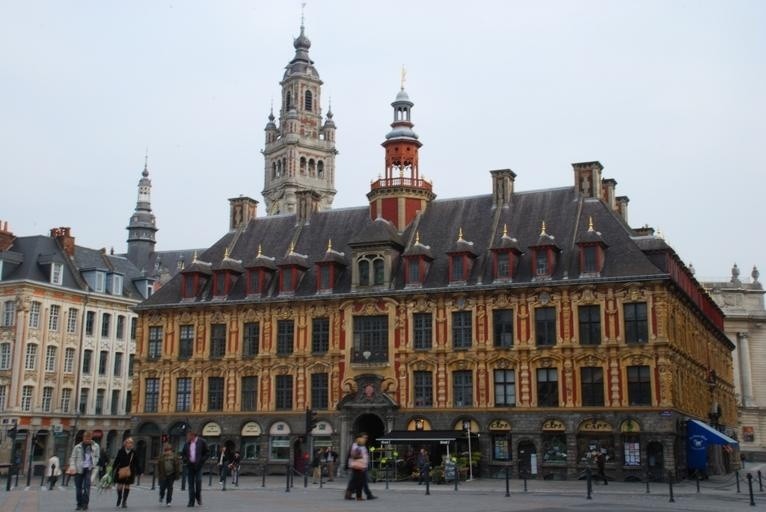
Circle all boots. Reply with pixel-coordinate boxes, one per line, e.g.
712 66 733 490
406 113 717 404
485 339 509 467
117 488 130 509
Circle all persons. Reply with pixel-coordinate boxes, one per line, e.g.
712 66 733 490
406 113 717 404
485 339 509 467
228 451 241 484
183 432 210 506
418 448 430 485
312 448 326 484
325 445 338 481
219 446 228 489
112 437 141 507
346 434 375 499
740 448 746 472
594 451 609 485
46 430 109 510
159 444 179 506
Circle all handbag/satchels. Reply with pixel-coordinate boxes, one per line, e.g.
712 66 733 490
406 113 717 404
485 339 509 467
119 466 130 479
348 456 369 473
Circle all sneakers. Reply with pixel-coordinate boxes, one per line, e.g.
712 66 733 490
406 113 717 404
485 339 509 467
159 498 172 506
75 502 89 511
187 498 203 507
345 494 378 501
